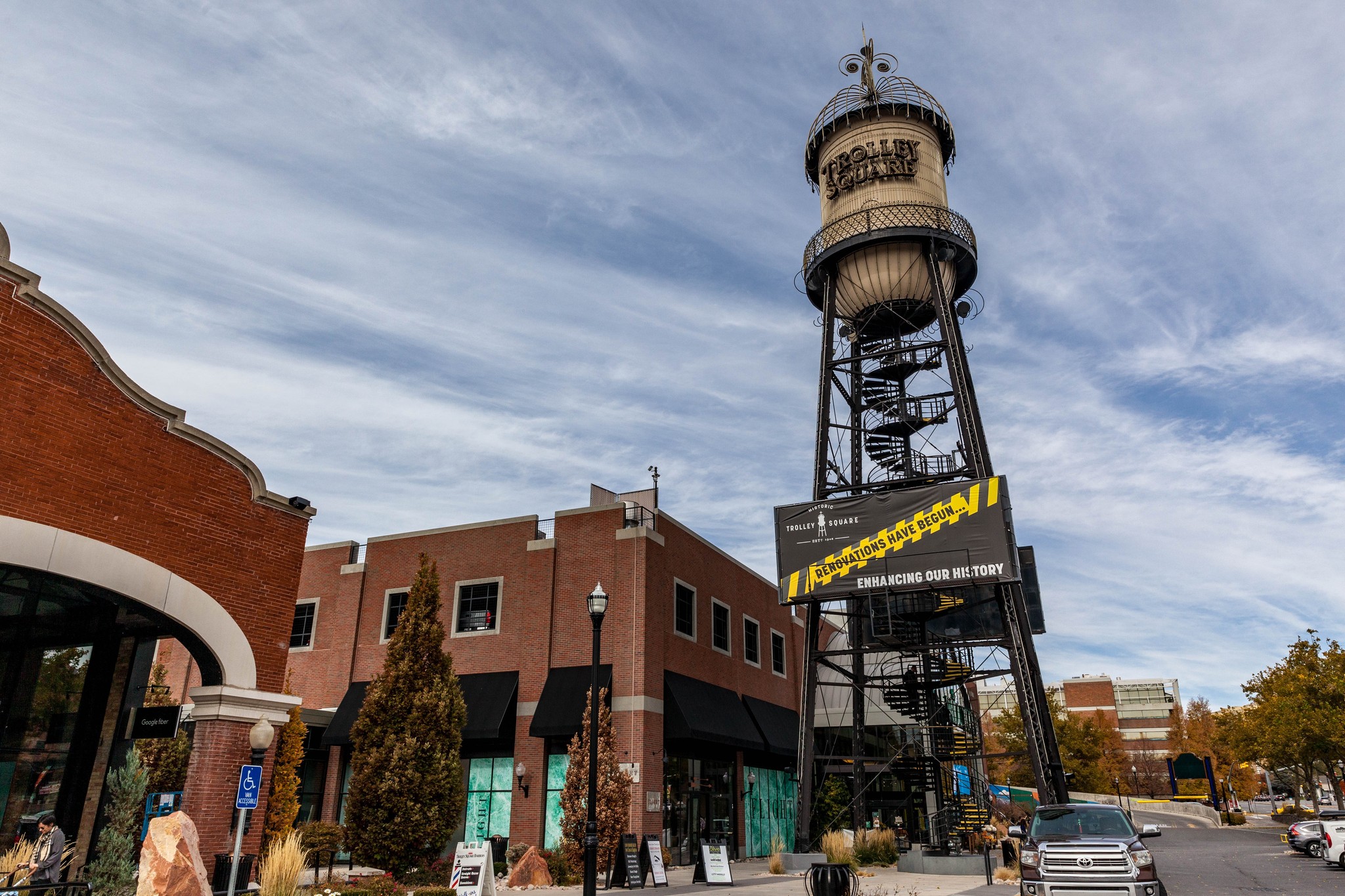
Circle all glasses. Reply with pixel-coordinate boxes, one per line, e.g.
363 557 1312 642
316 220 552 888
37 824 48 829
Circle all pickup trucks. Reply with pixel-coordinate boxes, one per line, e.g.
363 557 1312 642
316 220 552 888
1319 820 1345 870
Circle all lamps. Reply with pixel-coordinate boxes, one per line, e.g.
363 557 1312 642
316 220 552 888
289 496 310 511
653 748 669 764
138 685 170 695
740 772 756 801
515 762 529 798
230 713 274 836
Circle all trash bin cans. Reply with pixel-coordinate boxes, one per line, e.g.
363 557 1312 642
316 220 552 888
209 853 257 895
483 836 509 878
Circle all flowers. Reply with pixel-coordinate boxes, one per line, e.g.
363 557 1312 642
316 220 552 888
973 831 997 847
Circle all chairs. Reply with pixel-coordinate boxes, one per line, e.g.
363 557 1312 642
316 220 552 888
1096 817 1122 834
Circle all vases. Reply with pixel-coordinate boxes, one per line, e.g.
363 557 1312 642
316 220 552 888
976 846 990 855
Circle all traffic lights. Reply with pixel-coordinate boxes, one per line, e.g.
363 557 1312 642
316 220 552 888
1238 759 1248 769
1263 782 1266 787
1319 783 1323 788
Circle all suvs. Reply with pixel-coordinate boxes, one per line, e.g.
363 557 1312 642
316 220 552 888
1286 809 1345 858
1317 797 1332 806
1007 803 1168 896
1304 790 1312 801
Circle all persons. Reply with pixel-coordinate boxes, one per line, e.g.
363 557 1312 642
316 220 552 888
16 813 65 896
1016 816 1031 841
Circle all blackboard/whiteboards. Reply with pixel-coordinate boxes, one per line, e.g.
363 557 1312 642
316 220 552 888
610 833 645 887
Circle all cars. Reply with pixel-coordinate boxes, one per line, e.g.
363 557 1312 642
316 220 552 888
1271 804 1315 815
1254 794 1285 801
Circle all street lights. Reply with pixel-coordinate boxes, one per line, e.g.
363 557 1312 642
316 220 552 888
1218 777 1233 826
1132 765 1140 798
1006 777 1012 804
226 711 275 896
1115 777 1122 808
583 581 610 896
1337 759 1345 785
1315 760 1324 797
